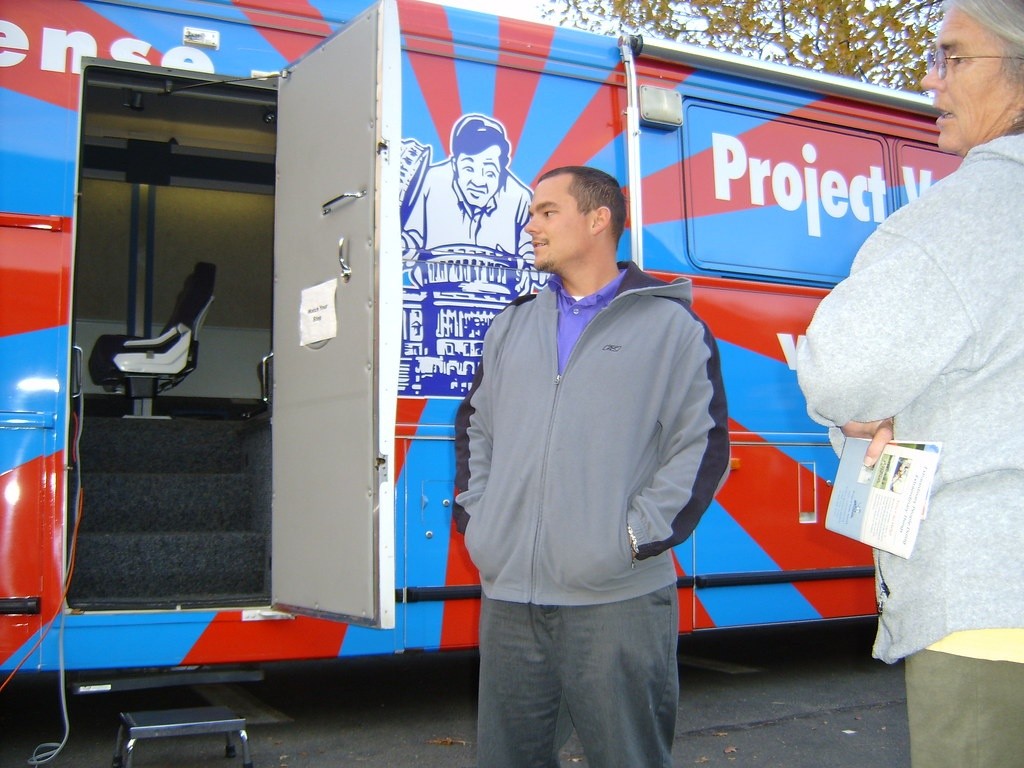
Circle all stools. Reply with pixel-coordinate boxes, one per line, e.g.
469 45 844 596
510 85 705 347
110 708 254 768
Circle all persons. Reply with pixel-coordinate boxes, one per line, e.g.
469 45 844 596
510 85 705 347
453 166 730 768
797 1 1023 768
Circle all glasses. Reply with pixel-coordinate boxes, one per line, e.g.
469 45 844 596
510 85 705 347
926 50 1024 80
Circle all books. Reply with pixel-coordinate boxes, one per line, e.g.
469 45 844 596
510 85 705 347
825 437 942 559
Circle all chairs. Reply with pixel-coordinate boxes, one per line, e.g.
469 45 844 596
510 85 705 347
91 259 219 420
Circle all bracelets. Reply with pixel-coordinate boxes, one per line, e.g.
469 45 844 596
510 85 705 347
628 527 640 553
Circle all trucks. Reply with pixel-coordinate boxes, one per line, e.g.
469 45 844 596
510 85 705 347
0 0 978 699
